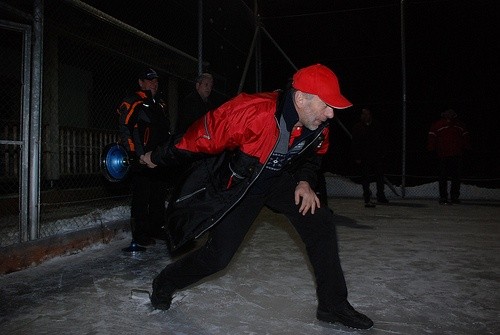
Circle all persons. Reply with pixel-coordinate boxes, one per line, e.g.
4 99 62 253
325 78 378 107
140 63 373 330
176 72 218 144
113 67 172 246
353 106 389 207
426 106 472 205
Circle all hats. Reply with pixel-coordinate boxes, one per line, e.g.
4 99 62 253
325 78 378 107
136 68 160 80
292 64 353 109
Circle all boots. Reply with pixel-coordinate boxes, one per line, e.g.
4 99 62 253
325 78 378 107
451 193 461 203
440 193 449 205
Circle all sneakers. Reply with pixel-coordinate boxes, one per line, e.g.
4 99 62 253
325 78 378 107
316 299 374 330
151 273 172 310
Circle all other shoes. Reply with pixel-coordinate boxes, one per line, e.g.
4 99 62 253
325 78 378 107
365 201 376 208
377 197 389 204
